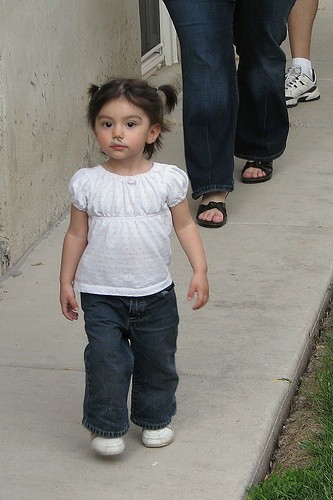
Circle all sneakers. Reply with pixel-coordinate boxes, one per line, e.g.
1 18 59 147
89 430 125 456
285 67 320 108
142 424 175 448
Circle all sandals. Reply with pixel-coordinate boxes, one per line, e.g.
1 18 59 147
196 189 230 228
241 159 273 183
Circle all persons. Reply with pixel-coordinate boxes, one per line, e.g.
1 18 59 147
159 0 297 228
59 77 210 457
285 0 321 108
